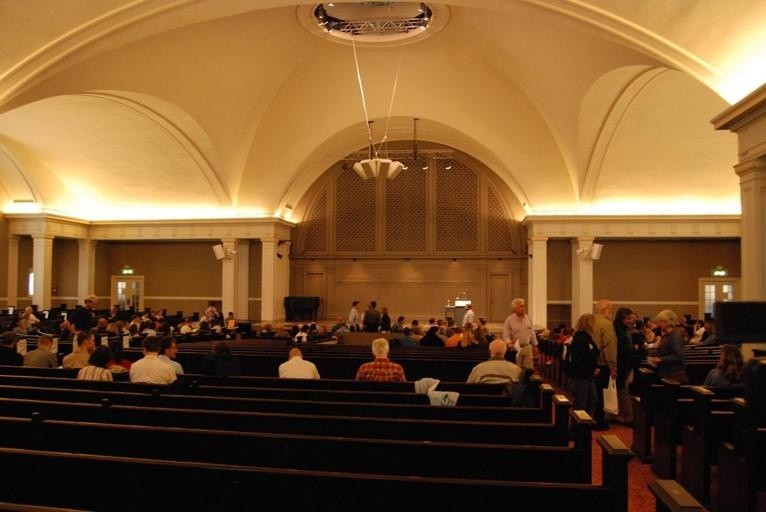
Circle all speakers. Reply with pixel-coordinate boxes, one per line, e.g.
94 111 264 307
210 244 226 261
591 243 602 261
351 159 406 181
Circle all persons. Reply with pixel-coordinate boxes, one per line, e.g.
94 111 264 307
264 299 538 384
0 294 242 384
554 299 744 431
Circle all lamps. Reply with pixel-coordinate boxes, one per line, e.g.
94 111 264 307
212 244 240 261
310 0 433 182
576 243 603 261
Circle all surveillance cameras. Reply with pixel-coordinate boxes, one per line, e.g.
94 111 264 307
575 247 585 256
232 249 238 256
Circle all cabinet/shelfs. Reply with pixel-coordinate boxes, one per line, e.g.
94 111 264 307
110 275 145 313
697 276 741 322
